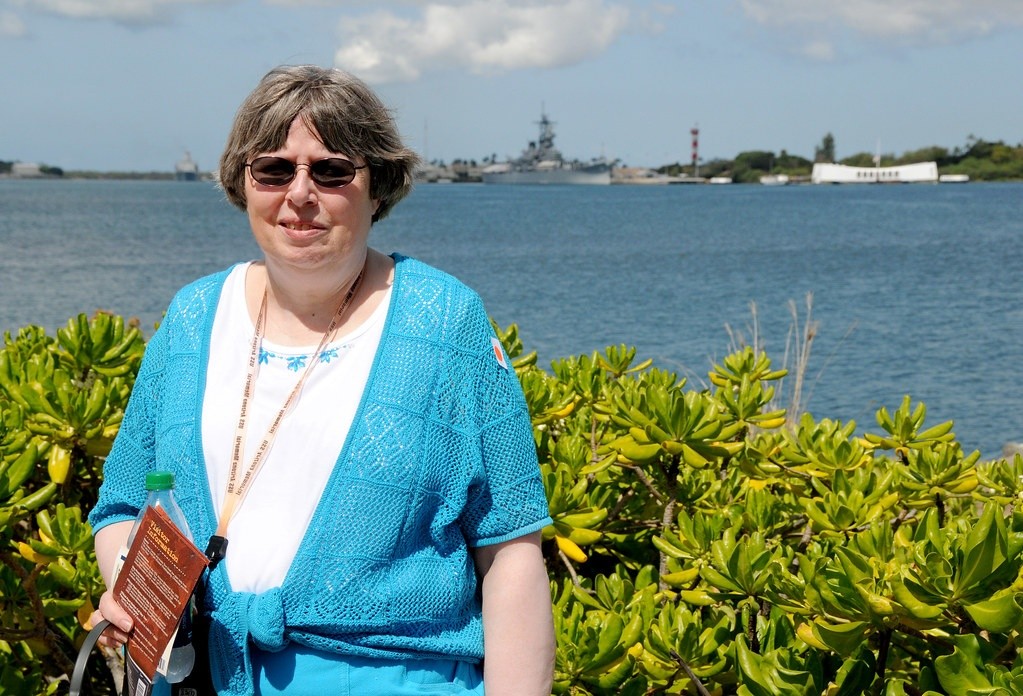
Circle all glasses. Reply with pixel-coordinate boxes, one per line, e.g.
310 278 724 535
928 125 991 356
242 155 371 188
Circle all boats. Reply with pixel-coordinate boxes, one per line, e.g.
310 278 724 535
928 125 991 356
759 172 790 188
477 114 615 187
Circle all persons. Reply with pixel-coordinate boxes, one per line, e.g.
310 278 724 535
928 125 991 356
88 66 554 696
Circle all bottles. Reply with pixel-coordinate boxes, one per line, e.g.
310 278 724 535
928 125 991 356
126 471 196 684
